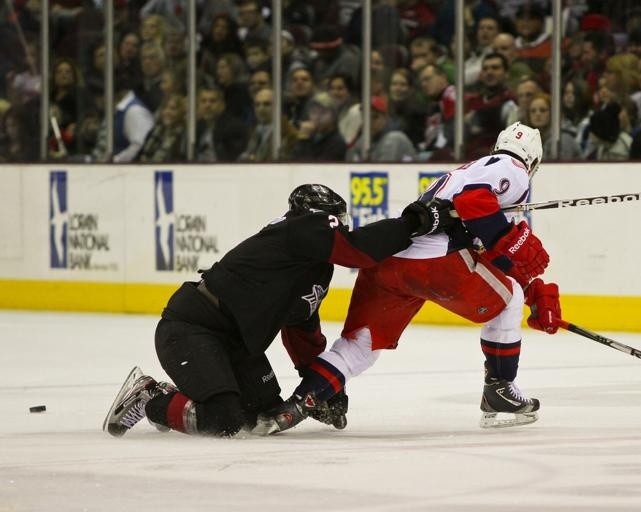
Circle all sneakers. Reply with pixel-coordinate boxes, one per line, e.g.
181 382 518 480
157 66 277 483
481 381 539 414
258 397 305 433
108 376 162 437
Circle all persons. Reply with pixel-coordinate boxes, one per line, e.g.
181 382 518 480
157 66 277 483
101 184 449 436
0 0 641 164
256 120 564 433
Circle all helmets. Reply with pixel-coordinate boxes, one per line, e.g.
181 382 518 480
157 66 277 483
288 184 346 226
492 121 543 180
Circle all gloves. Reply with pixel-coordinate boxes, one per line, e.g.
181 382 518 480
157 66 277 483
494 222 549 281
524 279 561 334
402 201 450 237
309 391 348 428
